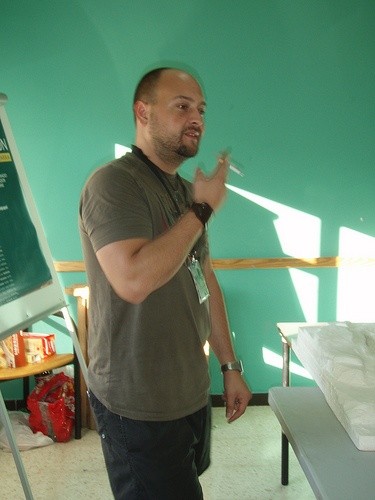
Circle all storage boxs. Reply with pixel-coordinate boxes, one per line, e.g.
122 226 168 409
23 333 56 357
0 330 25 367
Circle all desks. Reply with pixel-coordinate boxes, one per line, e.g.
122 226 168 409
276 322 375 486
267 386 375 500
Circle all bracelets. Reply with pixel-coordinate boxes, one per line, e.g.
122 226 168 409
221 359 244 375
190 201 214 228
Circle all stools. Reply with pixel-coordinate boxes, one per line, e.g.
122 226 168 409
0 352 82 440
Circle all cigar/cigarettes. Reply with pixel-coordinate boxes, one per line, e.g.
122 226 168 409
218 156 244 178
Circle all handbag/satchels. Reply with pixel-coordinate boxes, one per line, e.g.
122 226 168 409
27 372 75 443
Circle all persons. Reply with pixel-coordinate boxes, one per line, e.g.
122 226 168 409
79 68 251 500
